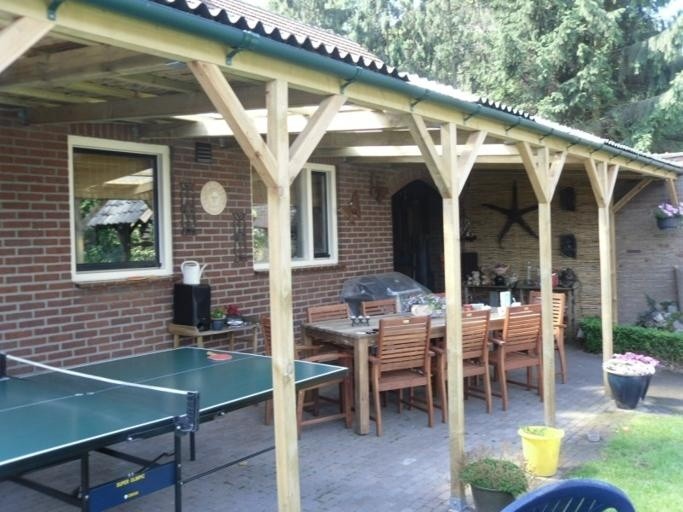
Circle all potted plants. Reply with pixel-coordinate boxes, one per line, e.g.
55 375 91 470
453 439 527 510
209 310 226 330
516 425 566 478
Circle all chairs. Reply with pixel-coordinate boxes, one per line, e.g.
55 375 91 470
308 303 356 411
352 315 435 437
262 315 351 433
466 304 541 411
408 309 492 423
528 291 565 384
360 298 398 317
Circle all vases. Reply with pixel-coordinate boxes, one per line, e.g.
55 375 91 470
602 365 653 410
226 317 243 325
657 217 677 231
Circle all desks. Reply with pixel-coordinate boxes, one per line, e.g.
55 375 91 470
464 282 578 344
302 307 505 434
166 322 259 354
0 344 349 512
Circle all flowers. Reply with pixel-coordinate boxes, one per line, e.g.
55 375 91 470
653 201 683 220
225 303 244 321
604 348 660 376
